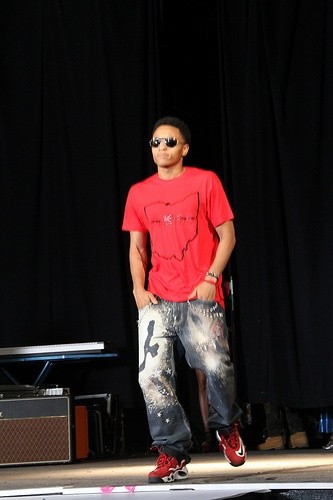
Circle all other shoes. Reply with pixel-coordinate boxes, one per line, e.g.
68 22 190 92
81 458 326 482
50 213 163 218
289 432 307 449
259 435 283 450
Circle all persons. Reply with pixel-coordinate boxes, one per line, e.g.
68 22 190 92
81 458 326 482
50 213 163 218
184 348 218 452
121 116 247 483
322 434 333 451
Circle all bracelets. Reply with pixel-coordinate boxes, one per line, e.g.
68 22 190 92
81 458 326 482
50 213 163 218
205 272 220 281
203 279 216 285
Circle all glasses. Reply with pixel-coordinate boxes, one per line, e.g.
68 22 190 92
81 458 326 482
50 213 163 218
149 136 186 148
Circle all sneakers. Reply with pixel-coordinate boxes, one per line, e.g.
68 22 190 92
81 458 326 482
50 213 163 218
217 421 247 467
147 445 189 482
